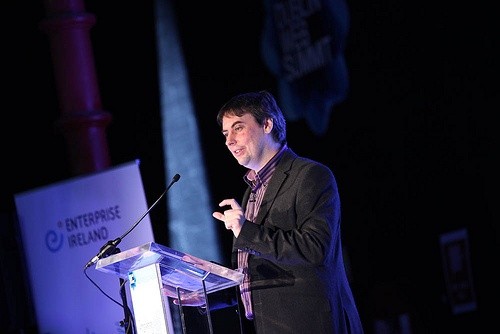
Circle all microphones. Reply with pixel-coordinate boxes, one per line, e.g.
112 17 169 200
86 173 180 267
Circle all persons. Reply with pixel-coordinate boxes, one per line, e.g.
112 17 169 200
173 90 365 334
